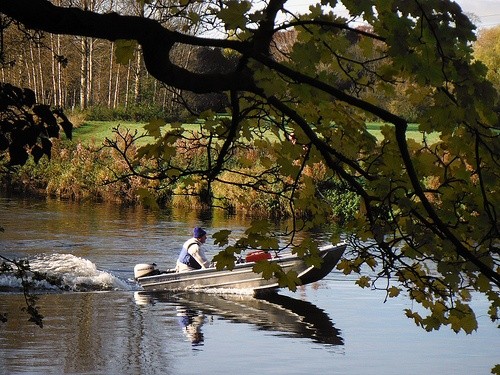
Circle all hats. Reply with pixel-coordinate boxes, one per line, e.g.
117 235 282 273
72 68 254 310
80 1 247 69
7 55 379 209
193 227 206 238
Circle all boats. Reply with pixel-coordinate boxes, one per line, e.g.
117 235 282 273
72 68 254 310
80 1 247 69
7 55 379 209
134 291 344 345
134 242 348 291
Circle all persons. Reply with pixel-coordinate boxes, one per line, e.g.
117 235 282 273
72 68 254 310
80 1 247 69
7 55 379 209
175 226 211 272
